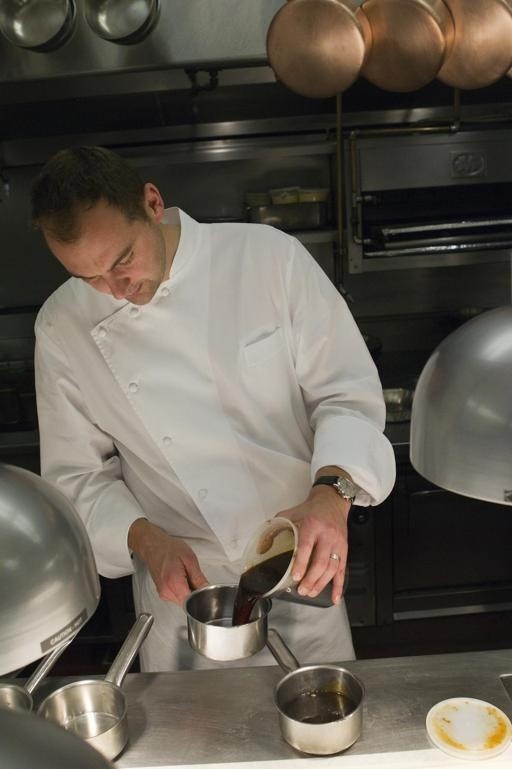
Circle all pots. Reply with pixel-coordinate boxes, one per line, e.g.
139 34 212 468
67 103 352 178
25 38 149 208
262 626 366 756
3 618 83 718
0 1 162 54
262 0 512 100
39 611 159 764
179 581 268 664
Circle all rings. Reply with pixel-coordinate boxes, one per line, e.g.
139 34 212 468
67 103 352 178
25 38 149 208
329 554 344 563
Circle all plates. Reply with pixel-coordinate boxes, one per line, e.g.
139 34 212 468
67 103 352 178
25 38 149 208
424 697 512 759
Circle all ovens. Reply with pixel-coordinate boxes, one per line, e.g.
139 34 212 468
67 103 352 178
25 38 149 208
332 121 510 659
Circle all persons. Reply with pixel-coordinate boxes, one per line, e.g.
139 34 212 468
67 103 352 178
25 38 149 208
29 144 397 676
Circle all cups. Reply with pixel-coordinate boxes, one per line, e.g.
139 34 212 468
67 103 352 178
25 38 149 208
240 516 338 607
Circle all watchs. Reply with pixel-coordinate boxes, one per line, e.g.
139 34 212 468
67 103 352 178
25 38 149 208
313 473 357 503
